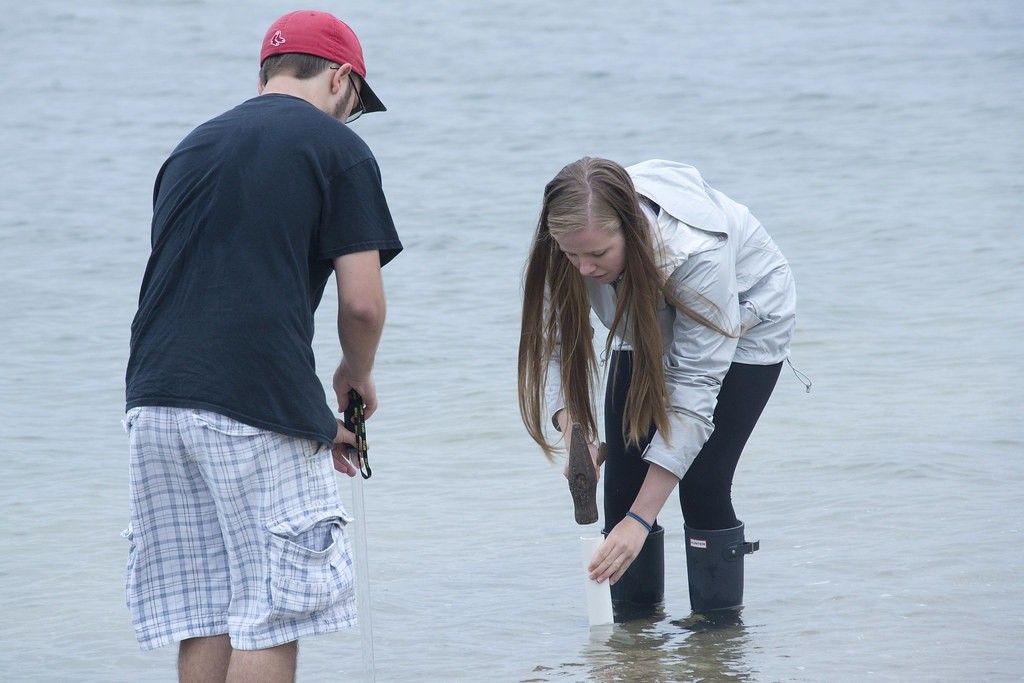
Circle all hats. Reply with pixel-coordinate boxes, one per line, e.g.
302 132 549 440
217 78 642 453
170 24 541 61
259 10 387 114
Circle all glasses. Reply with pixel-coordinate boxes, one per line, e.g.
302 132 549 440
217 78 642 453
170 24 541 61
330 67 365 125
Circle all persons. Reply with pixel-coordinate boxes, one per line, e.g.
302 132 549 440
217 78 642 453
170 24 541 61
518 156 796 623
122 10 403 683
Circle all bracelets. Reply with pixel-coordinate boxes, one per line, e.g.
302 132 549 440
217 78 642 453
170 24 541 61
626 512 652 531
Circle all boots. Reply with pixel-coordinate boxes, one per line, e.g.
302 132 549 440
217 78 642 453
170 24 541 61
603 527 663 626
683 523 760 628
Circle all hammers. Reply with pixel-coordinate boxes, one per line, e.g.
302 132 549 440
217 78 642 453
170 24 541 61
566 421 606 525
